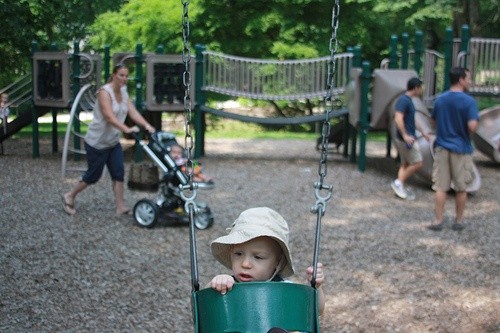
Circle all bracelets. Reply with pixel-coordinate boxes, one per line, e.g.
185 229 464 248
146 124 151 129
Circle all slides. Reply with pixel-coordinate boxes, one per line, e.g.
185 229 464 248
389 86 482 194
471 104 500 163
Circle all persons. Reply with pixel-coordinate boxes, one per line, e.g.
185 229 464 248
61 64 155 217
171 146 212 183
206 207 325 333
391 78 432 199
428 67 480 230
0 93 9 127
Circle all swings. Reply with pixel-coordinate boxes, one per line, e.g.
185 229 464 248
177 0 342 332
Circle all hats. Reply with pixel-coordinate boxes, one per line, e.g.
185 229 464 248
210 207 296 278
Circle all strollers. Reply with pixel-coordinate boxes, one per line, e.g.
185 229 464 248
123 123 218 229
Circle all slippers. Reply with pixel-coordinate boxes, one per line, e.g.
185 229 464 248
113 208 133 216
61 196 76 214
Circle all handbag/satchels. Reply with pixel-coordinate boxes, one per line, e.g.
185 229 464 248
128 157 159 192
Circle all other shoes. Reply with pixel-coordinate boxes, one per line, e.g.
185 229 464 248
452 219 467 230
391 180 406 198
425 220 443 231
